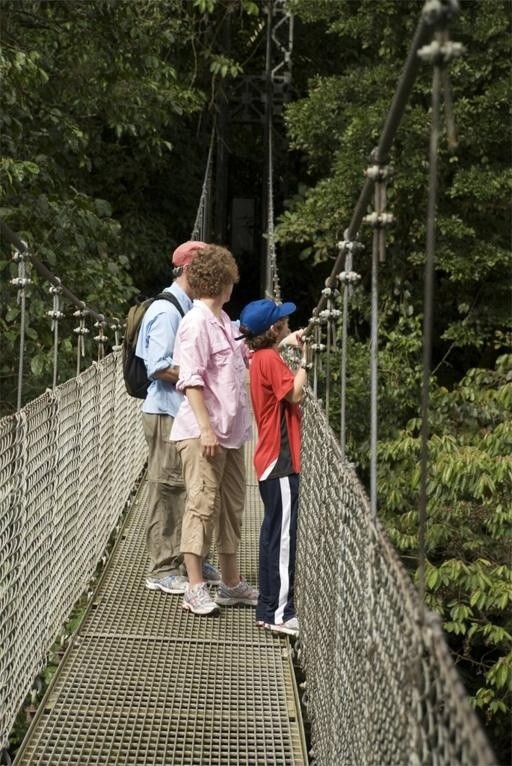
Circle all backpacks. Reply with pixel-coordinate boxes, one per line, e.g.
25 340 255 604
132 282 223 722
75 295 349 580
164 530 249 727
123 292 184 398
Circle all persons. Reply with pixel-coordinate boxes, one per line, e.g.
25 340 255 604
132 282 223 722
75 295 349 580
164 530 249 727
168 244 302 617
232 297 315 638
135 237 222 596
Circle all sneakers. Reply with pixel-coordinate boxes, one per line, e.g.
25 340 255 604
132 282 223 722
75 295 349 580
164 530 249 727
145 562 299 635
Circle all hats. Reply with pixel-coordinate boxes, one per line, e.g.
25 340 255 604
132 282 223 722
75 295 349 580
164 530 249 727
235 299 296 343
172 241 208 266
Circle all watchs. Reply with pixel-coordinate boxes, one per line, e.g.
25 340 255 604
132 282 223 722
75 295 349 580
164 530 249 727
300 362 313 371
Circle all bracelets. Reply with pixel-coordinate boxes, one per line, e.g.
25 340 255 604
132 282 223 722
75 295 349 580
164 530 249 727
282 338 288 350
